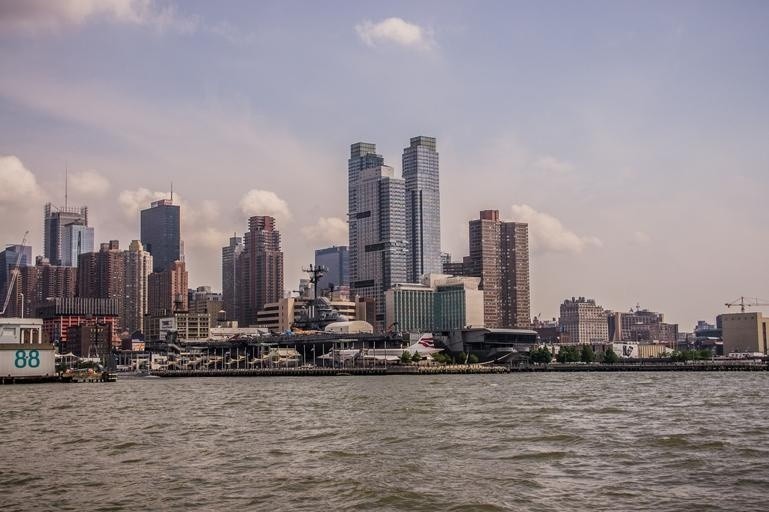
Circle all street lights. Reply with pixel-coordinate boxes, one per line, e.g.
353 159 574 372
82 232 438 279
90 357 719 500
94 321 111 358
19 293 24 319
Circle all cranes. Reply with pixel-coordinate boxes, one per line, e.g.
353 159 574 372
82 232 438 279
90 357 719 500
723 295 769 313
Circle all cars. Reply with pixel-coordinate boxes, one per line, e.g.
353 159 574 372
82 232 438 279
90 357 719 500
116 364 128 373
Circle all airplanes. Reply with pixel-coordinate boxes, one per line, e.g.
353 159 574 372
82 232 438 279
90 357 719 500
317 331 447 367
